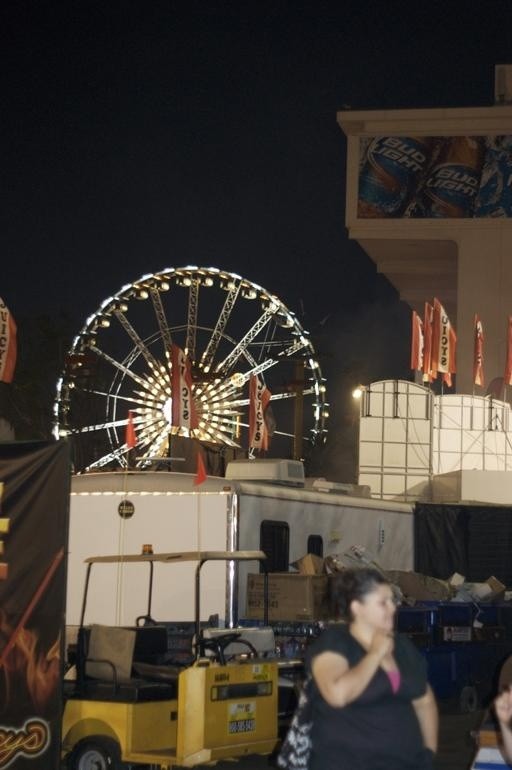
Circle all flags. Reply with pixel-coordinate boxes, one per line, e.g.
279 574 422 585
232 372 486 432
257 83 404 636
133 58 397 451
173 344 198 429
0 296 18 384
411 298 458 387
506 321 512 387
250 373 271 451
474 317 486 386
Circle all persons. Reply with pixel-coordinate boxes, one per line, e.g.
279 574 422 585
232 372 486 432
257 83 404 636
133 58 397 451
303 569 439 770
492 684 512 766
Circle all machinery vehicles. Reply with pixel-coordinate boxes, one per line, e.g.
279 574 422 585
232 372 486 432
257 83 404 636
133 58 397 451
60 549 283 770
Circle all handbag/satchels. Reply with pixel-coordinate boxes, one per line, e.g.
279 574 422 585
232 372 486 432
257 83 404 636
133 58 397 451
277 680 313 770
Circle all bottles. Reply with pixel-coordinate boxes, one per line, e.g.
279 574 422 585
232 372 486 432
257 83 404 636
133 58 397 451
359 137 512 217
